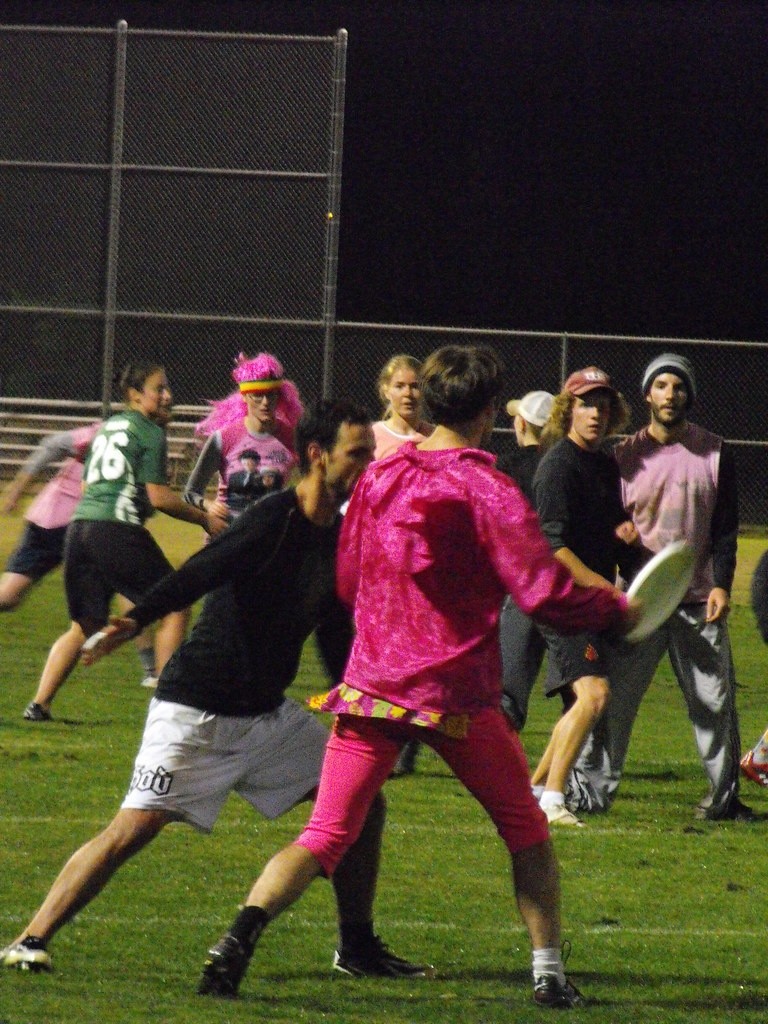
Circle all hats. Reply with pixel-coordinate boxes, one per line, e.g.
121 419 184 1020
642 353 697 402
507 390 556 427
565 366 616 395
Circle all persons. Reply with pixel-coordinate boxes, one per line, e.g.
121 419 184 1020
0 350 305 686
346 352 768 828
0 400 438 984
196 343 585 1008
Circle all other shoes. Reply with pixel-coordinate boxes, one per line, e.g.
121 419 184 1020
546 806 587 830
533 974 582 1008
740 751 768 787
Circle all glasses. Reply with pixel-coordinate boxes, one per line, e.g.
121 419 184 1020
249 391 280 403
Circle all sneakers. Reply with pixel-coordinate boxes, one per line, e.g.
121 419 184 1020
1 935 51 974
334 938 428 980
201 937 246 998
22 702 55 723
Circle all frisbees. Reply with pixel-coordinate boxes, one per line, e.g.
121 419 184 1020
622 539 698 643
